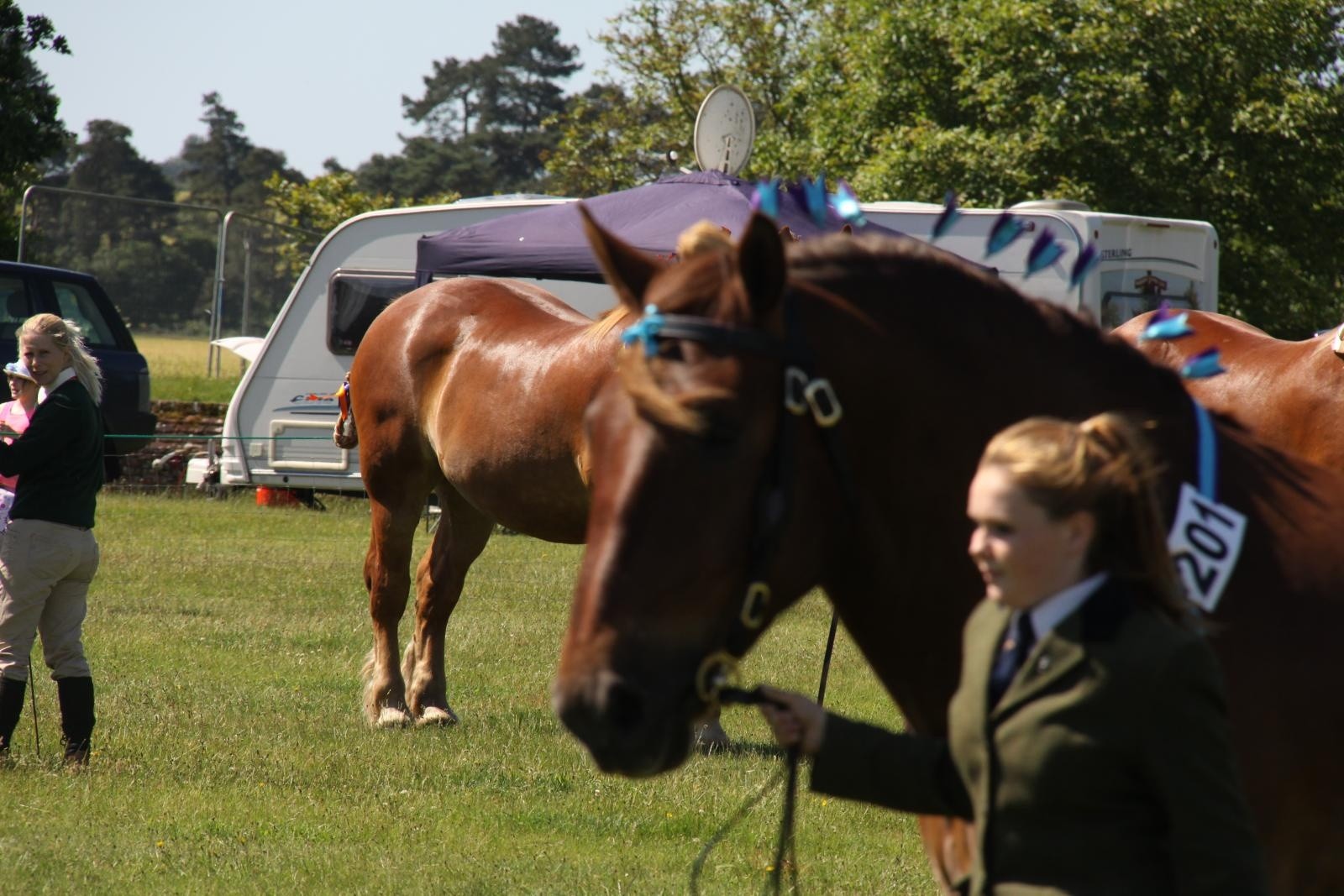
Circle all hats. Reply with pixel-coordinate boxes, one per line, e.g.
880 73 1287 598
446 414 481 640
4 358 39 387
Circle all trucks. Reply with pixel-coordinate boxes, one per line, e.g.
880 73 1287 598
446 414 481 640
185 192 1219 491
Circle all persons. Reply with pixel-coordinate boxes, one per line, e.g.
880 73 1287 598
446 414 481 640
0 313 101 771
762 415 1251 896
0 359 38 530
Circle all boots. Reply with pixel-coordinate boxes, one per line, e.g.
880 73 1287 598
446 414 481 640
0 676 27 753
54 677 95 771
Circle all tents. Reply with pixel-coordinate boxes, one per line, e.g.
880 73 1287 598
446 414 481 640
414 173 994 356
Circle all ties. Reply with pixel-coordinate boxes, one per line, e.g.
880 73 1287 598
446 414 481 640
1000 609 1035 696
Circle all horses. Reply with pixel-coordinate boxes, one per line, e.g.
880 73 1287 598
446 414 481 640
347 277 649 742
545 198 1344 895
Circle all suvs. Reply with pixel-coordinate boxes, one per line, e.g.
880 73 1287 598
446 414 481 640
0 261 158 482
